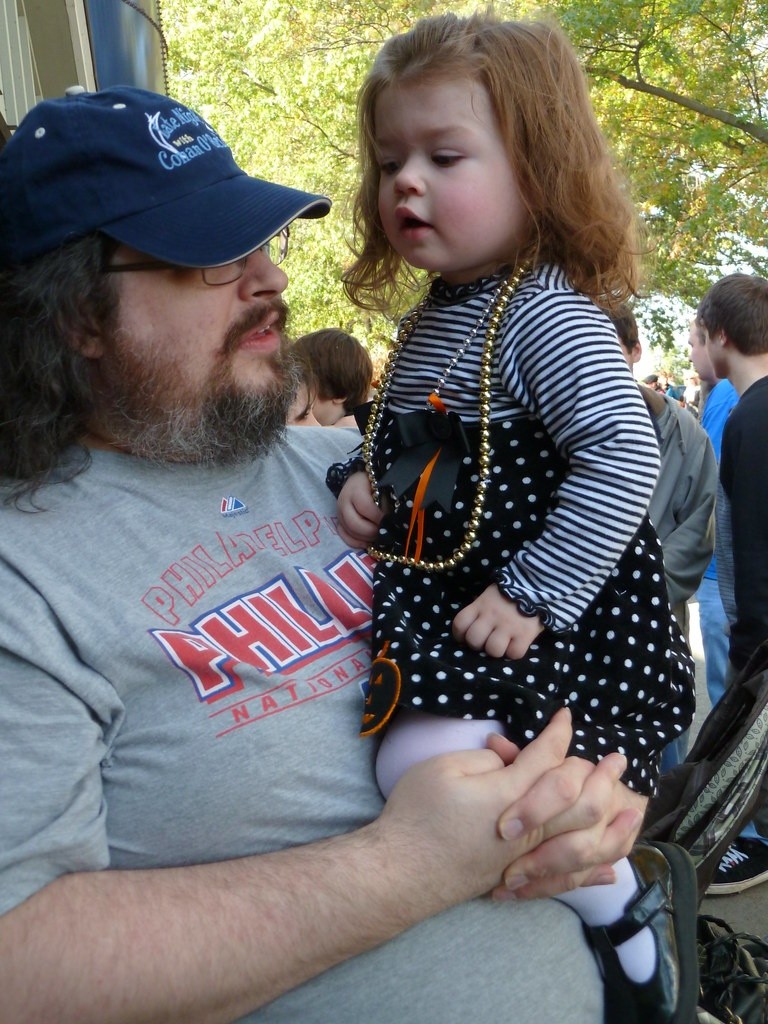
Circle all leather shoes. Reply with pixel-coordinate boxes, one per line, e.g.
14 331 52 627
585 840 700 1024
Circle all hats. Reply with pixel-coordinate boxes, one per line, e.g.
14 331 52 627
0 85 333 270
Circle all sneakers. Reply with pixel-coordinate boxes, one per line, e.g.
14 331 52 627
704 838 768 896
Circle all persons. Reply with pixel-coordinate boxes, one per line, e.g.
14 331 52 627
294 328 373 428
0 83 645 1024
323 12 698 1024
286 375 323 427
604 268 768 897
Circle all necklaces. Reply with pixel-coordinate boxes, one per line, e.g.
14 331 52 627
362 241 531 568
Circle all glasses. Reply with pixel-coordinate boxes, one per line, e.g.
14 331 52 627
108 225 291 284
